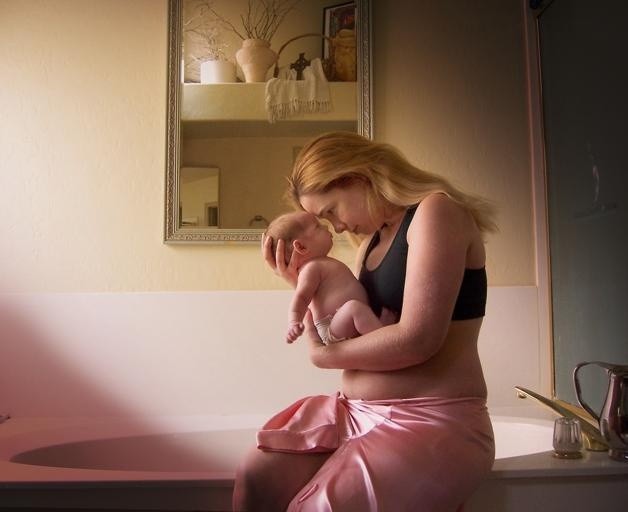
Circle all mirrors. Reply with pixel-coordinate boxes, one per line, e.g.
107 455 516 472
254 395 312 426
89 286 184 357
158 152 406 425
178 166 220 230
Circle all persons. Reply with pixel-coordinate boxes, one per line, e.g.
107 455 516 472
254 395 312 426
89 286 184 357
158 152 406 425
264 207 402 340
228 134 498 511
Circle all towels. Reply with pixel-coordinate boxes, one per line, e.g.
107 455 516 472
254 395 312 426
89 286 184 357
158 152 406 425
265 58 332 123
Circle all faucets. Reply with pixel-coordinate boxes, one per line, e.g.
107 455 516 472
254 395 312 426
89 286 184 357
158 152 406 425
512 385 609 450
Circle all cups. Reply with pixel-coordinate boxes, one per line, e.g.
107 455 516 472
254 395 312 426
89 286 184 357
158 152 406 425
553 418 583 460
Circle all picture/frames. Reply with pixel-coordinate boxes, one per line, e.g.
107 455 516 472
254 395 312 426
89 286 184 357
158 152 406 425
322 1 356 64
163 0 374 249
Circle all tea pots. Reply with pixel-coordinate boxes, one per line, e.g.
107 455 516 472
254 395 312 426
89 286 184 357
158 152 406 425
573 361 628 461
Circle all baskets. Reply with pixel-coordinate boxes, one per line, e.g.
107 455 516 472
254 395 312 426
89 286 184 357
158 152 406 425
272 32 338 82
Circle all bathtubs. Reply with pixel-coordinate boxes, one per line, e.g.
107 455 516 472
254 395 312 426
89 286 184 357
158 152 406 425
0 415 627 512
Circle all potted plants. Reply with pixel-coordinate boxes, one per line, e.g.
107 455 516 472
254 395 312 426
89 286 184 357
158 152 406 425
184 0 299 83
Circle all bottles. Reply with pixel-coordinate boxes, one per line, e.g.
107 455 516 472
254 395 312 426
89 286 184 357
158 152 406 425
292 53 309 81
